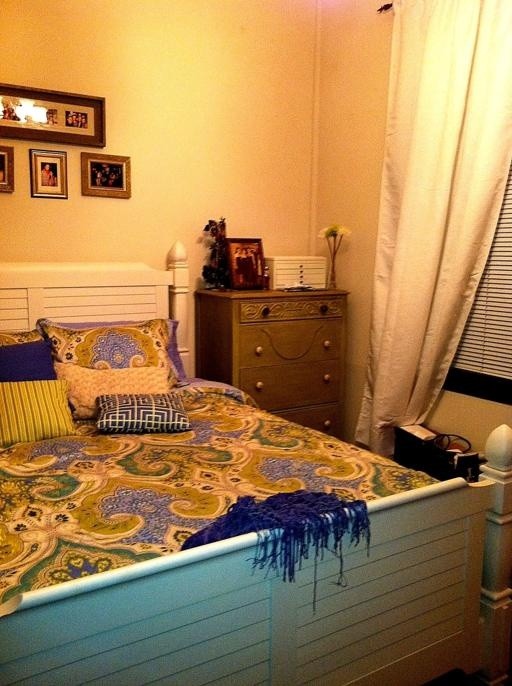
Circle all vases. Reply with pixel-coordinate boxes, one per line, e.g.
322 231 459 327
327 262 337 289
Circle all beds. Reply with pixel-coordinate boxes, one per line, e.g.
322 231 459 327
0 241 511 686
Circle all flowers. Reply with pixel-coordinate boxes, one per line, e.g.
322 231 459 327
318 225 351 281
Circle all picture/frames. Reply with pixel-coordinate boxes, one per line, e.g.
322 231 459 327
29 148 68 199
0 145 15 192
80 152 131 199
0 83 106 148
226 238 264 290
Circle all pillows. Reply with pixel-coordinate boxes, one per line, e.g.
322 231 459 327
0 327 43 347
54 360 170 419
0 340 57 381
95 393 193 434
0 380 81 445
36 318 189 389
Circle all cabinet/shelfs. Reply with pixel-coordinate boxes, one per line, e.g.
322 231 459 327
195 288 350 439
265 256 327 291
